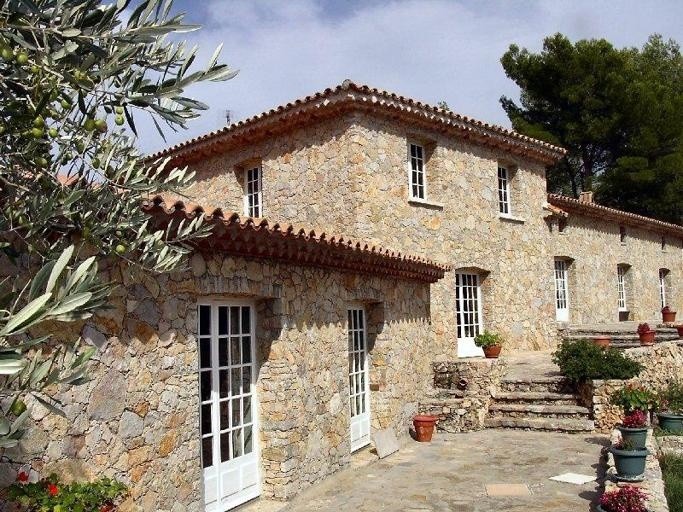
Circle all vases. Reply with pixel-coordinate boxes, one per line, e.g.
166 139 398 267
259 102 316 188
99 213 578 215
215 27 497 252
607 379 682 480
595 503 616 512
413 414 439 441
593 336 610 348
675 324 682 338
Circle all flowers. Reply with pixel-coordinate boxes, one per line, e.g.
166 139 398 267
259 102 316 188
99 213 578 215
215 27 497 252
599 484 647 511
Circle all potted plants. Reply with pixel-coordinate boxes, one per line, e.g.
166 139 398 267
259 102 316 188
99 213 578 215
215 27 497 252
661 306 676 323
635 323 655 345
474 330 504 358
